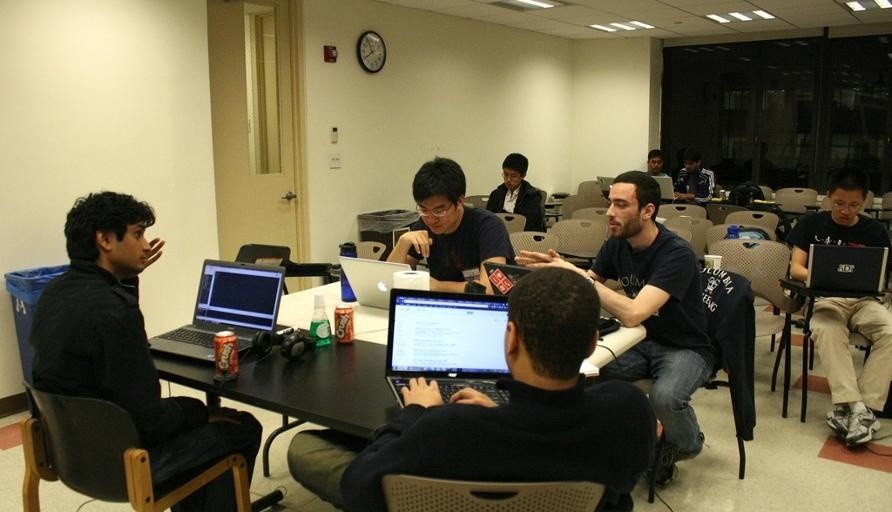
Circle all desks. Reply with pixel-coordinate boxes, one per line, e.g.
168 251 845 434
779 270 892 423
545 195 564 216
276 279 647 380
599 178 696 204
148 321 510 442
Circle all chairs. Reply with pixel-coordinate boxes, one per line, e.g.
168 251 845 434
571 207 612 221
463 195 491 210
761 183 772 201
721 210 779 243
822 190 830 210
381 472 606 512
774 187 818 215
548 219 608 266
881 190 892 218
660 215 712 263
562 180 609 219
509 231 560 268
654 204 707 220
494 212 527 231
21 381 255 512
630 265 752 504
327 240 387 278
709 239 791 418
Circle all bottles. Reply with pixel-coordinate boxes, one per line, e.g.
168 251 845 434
334 304 355 343
214 330 239 376
727 225 739 239
309 293 332 346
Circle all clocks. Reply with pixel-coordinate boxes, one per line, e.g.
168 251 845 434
357 30 389 74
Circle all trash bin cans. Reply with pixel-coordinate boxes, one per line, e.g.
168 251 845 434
3 265 73 419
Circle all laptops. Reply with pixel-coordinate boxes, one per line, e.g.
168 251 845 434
340 254 413 311
804 244 888 299
145 258 286 365
482 261 621 338
388 288 511 410
651 175 679 202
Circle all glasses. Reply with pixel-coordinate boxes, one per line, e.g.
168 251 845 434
415 203 453 217
832 200 862 210
501 172 522 180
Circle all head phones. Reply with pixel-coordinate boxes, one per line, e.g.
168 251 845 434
251 330 317 362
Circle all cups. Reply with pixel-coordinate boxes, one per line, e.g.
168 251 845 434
703 254 724 269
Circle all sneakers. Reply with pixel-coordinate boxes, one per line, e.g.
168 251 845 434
646 432 705 469
642 463 679 488
825 405 849 440
845 407 881 448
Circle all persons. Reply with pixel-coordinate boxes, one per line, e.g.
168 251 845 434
645 149 669 178
674 149 715 204
338 268 656 512
33 192 261 511
792 177 892 443
484 153 547 234
388 156 515 295
517 170 716 456
